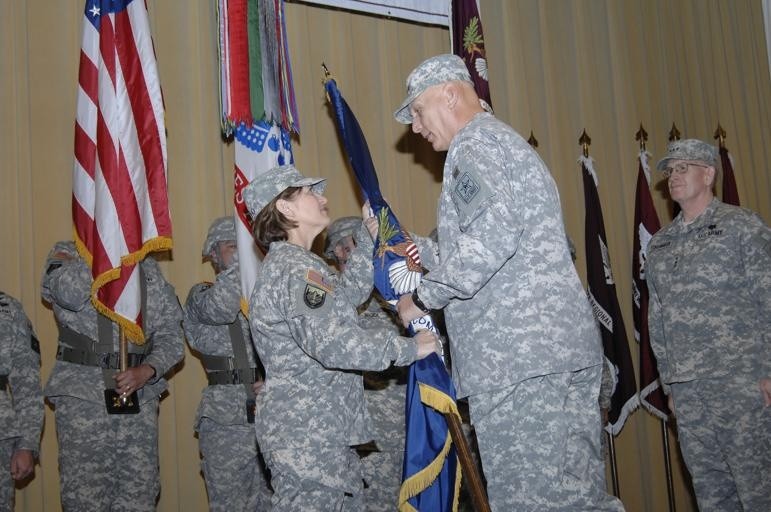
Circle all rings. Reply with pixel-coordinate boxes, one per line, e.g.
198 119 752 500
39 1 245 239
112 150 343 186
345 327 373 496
127 384 130 389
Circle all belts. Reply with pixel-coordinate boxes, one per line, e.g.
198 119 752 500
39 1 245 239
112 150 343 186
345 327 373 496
205 368 266 385
54 344 144 369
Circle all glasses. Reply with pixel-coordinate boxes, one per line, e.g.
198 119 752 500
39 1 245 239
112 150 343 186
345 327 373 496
661 163 708 174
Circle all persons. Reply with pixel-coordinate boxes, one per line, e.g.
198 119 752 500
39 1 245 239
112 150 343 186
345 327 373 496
42 241 184 512
185 215 274 512
646 140 771 512
390 54 626 512
327 217 375 277
1 291 45 512
242 165 442 512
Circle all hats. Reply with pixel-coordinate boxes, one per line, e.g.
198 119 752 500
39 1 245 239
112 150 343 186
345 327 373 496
241 163 328 224
392 53 475 126
657 138 715 172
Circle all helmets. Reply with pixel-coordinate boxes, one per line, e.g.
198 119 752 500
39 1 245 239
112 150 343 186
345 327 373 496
322 215 363 268
200 216 237 269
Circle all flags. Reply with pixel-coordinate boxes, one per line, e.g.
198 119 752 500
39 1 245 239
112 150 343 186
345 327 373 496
630 157 690 428
668 125 681 143
581 157 640 436
529 130 538 147
716 146 743 209
72 1 173 344
216 1 302 321
321 63 464 512
450 0 493 112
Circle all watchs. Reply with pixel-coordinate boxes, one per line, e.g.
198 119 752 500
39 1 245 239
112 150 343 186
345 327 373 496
412 289 432 314
